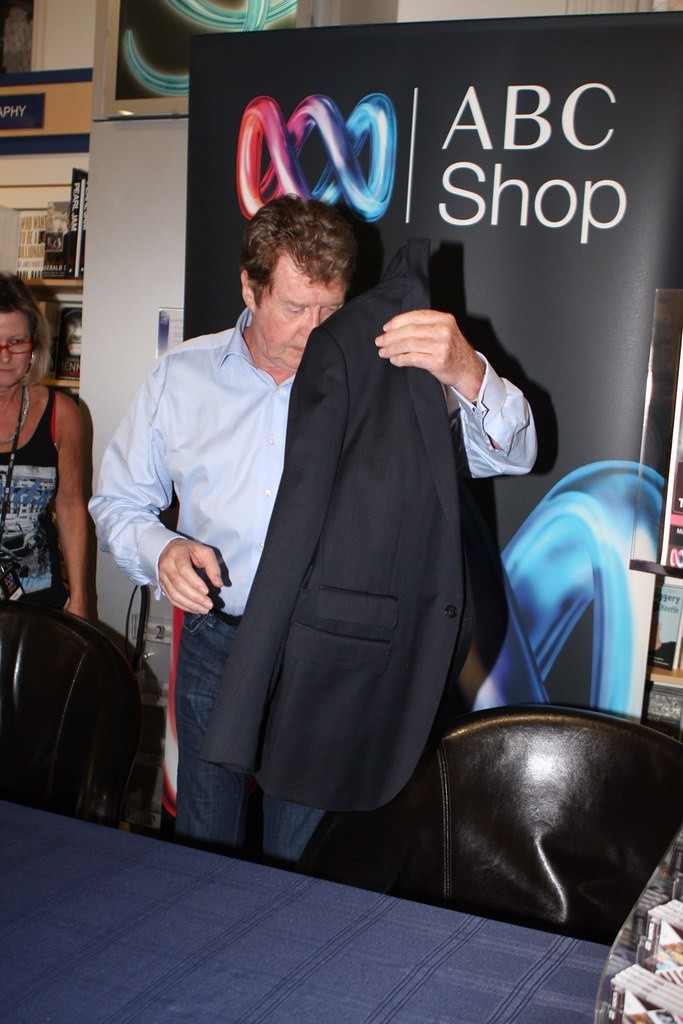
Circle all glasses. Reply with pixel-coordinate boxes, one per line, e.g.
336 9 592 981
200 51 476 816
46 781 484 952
1 341 33 354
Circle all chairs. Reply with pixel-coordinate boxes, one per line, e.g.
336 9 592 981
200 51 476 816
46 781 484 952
0 599 165 831
431 703 683 945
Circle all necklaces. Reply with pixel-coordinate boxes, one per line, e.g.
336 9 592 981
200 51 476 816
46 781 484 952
0 386 30 444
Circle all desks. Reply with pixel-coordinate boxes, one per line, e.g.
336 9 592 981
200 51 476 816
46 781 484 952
1 798 616 1024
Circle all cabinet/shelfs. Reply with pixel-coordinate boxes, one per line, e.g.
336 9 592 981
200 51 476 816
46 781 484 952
0 68 93 388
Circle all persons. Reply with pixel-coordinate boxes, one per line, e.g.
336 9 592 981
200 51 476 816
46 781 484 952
89 195 538 867
0 270 87 619
